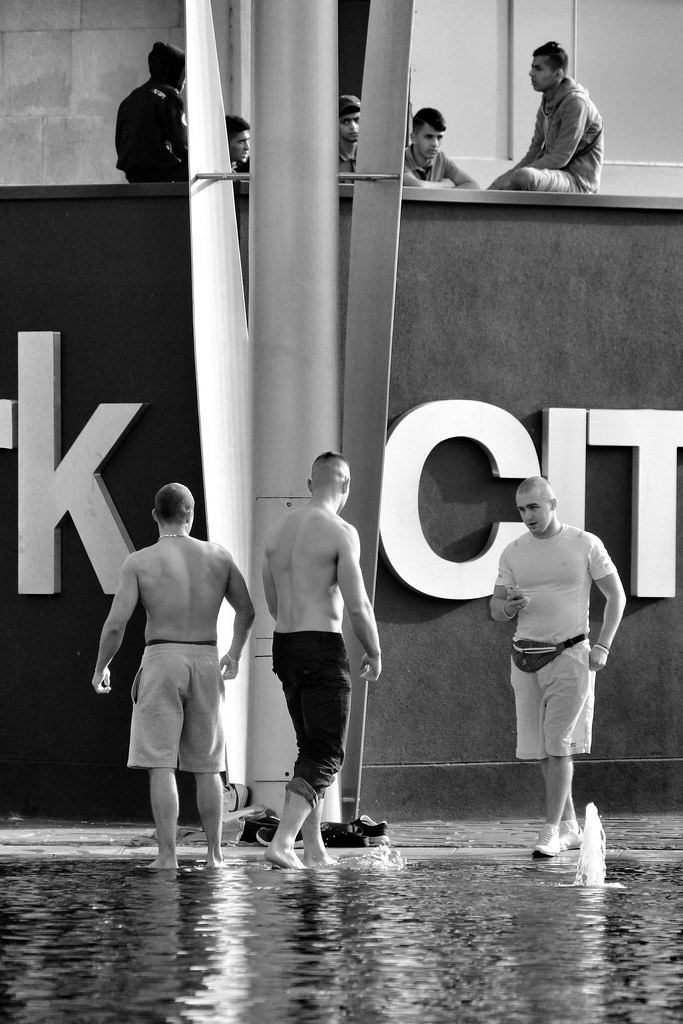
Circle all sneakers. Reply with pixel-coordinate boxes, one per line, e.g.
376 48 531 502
532 826 561 857
559 822 584 851
350 814 390 843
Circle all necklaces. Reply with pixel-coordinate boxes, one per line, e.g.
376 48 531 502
158 533 190 542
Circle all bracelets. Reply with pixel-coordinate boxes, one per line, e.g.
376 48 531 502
594 642 610 655
226 652 239 664
503 600 516 619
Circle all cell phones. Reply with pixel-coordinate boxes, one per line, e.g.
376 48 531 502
504 584 525 599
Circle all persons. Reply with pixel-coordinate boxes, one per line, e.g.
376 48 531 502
489 475 628 858
225 113 250 173
92 482 256 869
401 108 481 189
484 40 604 193
114 41 189 182
261 451 383 869
336 94 362 182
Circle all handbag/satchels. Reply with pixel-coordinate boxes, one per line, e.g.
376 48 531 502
510 634 589 672
